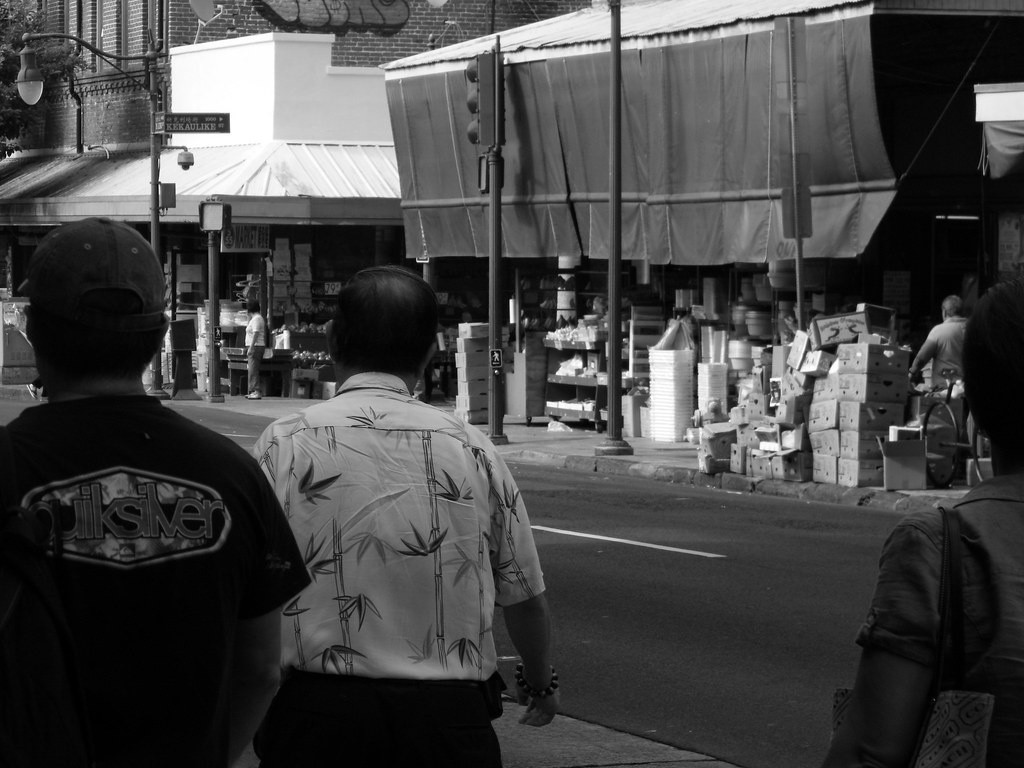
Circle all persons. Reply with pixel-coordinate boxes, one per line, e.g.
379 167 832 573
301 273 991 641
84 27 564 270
908 296 970 477
0 218 309 767
822 266 1023 768
251 262 562 768
243 298 267 399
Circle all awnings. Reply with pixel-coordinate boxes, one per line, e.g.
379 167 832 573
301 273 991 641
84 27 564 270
1 145 403 226
975 82 1024 182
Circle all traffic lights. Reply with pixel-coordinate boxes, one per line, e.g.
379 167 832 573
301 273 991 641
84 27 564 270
467 54 506 147
479 155 504 193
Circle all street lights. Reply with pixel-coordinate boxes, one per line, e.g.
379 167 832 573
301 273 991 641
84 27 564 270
18 29 194 402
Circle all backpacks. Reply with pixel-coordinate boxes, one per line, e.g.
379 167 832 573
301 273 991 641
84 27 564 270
0 426 96 768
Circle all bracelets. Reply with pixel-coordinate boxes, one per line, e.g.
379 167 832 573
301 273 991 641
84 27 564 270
908 367 917 375
515 662 559 698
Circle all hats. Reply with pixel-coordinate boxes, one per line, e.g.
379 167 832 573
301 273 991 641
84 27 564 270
18 217 167 332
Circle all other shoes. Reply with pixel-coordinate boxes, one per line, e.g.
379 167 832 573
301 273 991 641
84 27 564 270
248 391 260 399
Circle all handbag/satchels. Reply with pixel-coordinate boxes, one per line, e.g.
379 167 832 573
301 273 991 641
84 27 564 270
830 506 994 768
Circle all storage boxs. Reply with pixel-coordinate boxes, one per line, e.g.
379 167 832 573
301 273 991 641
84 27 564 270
647 347 693 443
289 368 340 400
697 305 927 489
454 318 491 425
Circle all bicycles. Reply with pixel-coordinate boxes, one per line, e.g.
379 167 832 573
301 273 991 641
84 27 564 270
923 366 995 486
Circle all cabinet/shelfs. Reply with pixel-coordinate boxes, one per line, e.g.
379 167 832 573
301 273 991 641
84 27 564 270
543 332 628 429
